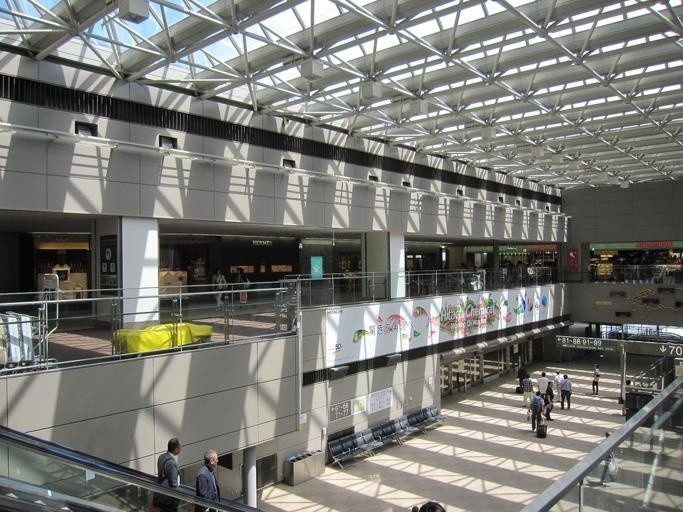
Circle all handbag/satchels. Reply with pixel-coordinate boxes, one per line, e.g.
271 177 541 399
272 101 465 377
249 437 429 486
152 489 181 510
515 387 524 394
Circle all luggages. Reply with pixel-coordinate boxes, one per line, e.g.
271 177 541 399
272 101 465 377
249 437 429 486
536 414 547 438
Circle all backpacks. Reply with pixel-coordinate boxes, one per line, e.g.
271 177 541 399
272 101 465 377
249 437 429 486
530 396 542 412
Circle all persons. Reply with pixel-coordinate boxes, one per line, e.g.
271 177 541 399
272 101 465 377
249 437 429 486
591 363 601 395
211 268 228 310
515 360 572 429
151 438 183 512
586 249 679 284
598 432 618 487
410 501 446 512
194 449 220 512
338 260 555 296
621 380 633 415
234 267 249 308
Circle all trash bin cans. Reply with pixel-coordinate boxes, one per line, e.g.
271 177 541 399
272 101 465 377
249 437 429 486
283 448 325 486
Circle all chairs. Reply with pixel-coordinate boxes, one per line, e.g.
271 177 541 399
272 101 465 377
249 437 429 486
326 404 447 469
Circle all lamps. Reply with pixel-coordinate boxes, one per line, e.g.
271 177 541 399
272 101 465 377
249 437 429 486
410 100 630 189
362 82 382 99
119 0 149 24
301 60 323 78
